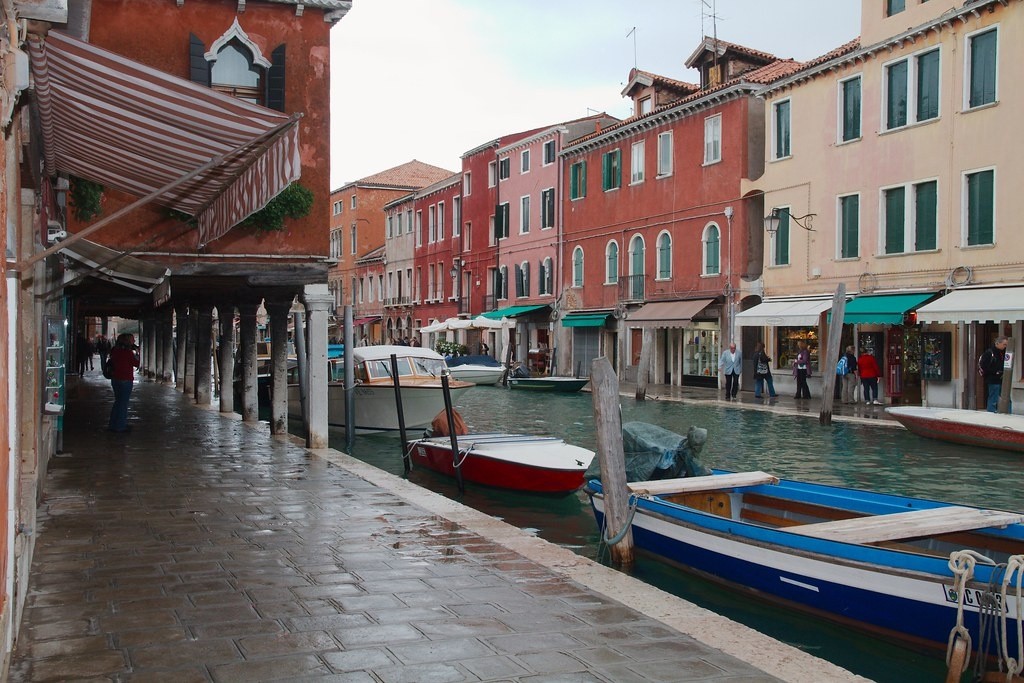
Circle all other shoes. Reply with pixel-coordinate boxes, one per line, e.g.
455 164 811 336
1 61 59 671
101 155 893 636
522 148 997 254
866 402 870 405
873 401 882 405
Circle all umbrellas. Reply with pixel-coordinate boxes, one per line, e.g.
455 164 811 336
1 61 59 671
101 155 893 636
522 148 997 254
420 315 516 343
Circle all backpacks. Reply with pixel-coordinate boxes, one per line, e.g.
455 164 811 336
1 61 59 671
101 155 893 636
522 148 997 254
978 350 994 376
103 360 113 378
835 355 849 376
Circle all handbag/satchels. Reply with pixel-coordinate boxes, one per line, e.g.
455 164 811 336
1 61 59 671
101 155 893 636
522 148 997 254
757 354 768 375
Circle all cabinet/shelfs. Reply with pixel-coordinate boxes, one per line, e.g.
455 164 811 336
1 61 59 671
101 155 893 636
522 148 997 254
45 343 66 416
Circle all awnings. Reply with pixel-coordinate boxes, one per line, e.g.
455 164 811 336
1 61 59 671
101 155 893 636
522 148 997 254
17 26 306 303
624 299 715 329
916 287 1024 324
561 314 611 326
470 305 549 320
55 231 171 307
340 317 383 332
734 299 850 326
827 293 936 325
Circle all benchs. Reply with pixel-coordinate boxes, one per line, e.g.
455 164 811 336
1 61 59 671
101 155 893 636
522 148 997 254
626 469 781 495
775 505 1024 545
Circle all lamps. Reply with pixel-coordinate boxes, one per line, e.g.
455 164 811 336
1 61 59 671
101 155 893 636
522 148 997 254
449 259 467 280
764 207 817 238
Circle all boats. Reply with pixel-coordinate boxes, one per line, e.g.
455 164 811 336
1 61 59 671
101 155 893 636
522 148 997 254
406 432 596 494
507 377 590 392
444 355 508 386
584 420 1024 679
885 406 1024 454
233 345 477 432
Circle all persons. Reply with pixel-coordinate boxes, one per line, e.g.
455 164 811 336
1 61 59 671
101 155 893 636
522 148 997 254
76 332 86 377
858 349 884 405
390 337 402 345
109 333 140 433
329 337 343 344
480 339 489 355
793 341 812 399
86 338 96 371
361 335 369 346
718 343 742 401
841 346 858 404
404 337 410 346
97 335 111 371
413 337 421 347
980 336 1008 412
753 342 779 398
